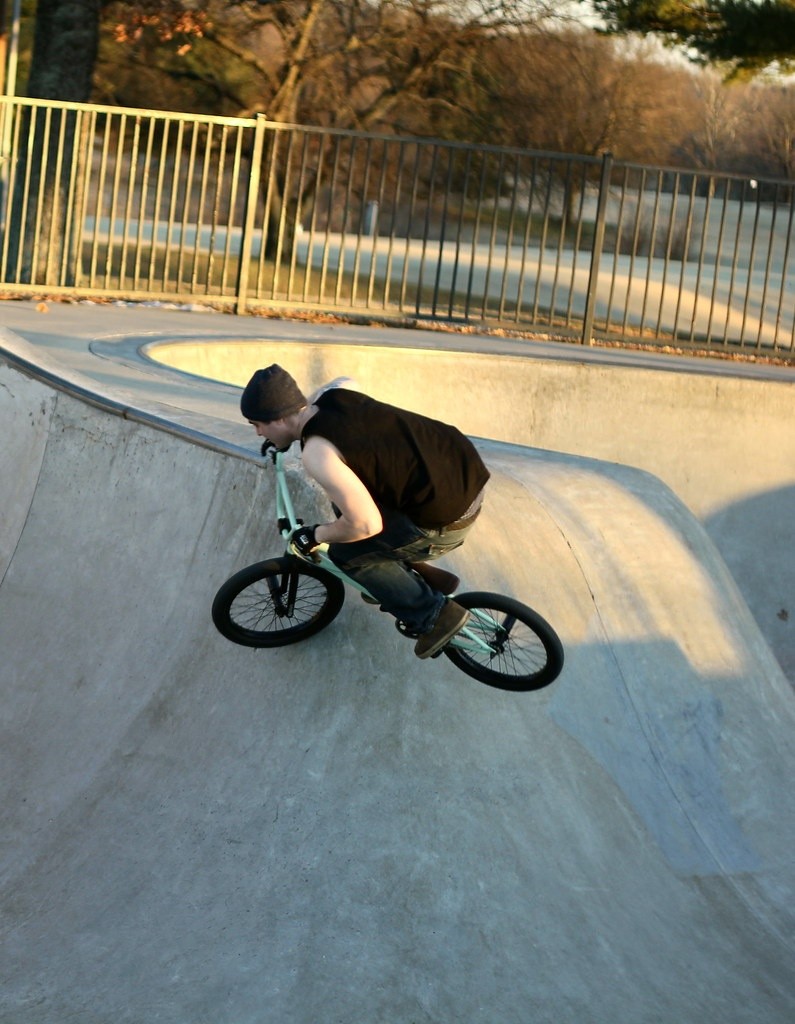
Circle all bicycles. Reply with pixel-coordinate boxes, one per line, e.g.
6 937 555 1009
210 447 565 693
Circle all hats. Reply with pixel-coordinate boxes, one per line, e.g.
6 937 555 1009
240 363 307 421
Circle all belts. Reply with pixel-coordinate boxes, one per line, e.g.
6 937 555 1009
434 505 482 532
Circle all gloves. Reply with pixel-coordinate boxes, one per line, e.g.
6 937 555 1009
262 437 291 457
289 523 323 565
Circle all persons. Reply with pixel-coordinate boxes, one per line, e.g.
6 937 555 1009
240 364 492 660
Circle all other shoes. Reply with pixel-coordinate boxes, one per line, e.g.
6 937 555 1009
414 596 470 661
